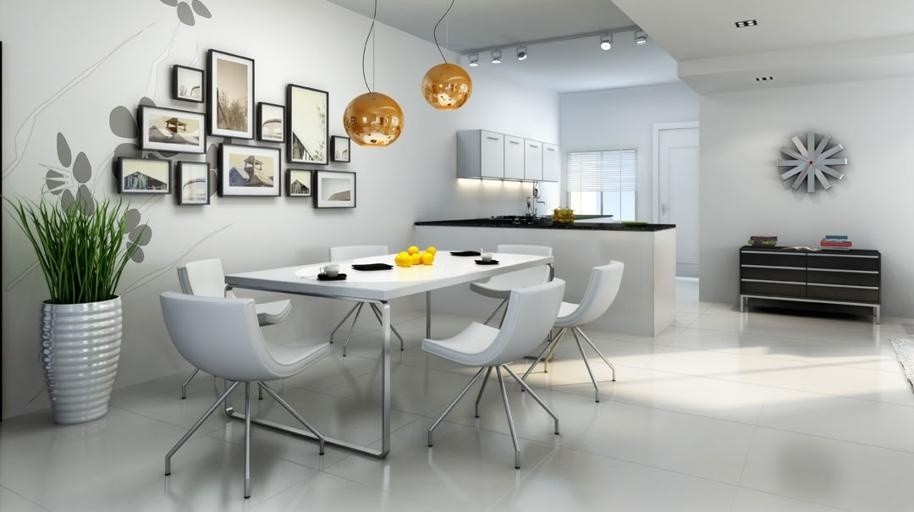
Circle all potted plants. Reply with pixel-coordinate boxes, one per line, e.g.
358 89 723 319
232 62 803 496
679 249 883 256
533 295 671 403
1 176 151 426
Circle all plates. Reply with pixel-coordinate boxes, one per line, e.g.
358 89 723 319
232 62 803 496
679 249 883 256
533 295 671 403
475 260 499 264
352 263 392 270
318 273 346 280
450 251 481 256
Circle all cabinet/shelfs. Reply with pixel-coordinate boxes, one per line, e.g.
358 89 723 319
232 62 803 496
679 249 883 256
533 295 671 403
739 245 882 324
456 129 562 182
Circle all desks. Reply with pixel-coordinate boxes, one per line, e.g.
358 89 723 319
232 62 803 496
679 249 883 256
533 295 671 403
223 249 555 460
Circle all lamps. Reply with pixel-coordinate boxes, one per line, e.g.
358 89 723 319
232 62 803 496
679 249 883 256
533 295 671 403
516 45 528 61
634 28 647 45
421 0 473 112
490 48 502 64
600 33 613 51
468 53 480 67
343 0 405 148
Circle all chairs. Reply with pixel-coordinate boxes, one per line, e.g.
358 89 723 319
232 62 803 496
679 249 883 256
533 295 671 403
421 277 566 470
159 291 330 499
177 257 293 400
329 244 404 357
469 244 553 330
521 259 625 403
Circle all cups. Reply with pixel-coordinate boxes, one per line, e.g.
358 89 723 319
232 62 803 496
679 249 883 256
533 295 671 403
482 250 492 261
320 265 339 276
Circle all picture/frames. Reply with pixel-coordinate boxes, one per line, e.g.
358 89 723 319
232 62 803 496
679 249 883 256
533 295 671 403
216 142 282 197
176 160 211 206
172 64 204 104
137 104 207 154
118 156 173 194
286 83 356 209
256 102 285 143
206 49 255 140
